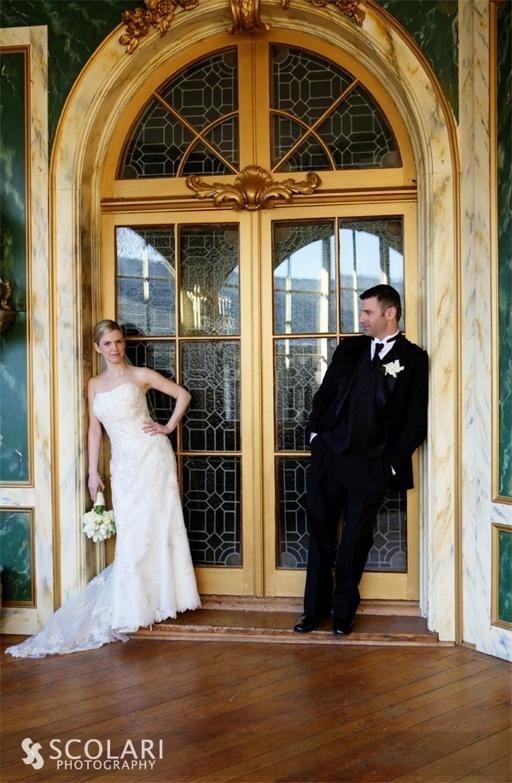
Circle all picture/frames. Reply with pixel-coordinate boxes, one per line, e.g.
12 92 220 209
0 45 33 486
490 524 511 632
488 1 512 507
1 506 36 609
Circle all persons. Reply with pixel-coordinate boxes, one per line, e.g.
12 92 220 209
294 285 427 636
82 320 202 624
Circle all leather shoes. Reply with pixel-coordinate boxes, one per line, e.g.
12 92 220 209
332 613 351 635
294 611 324 632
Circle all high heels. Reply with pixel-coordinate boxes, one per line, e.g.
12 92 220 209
117 624 154 634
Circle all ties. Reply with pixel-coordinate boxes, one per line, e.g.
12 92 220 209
374 343 383 367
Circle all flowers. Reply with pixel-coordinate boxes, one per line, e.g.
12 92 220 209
83 472 116 543
